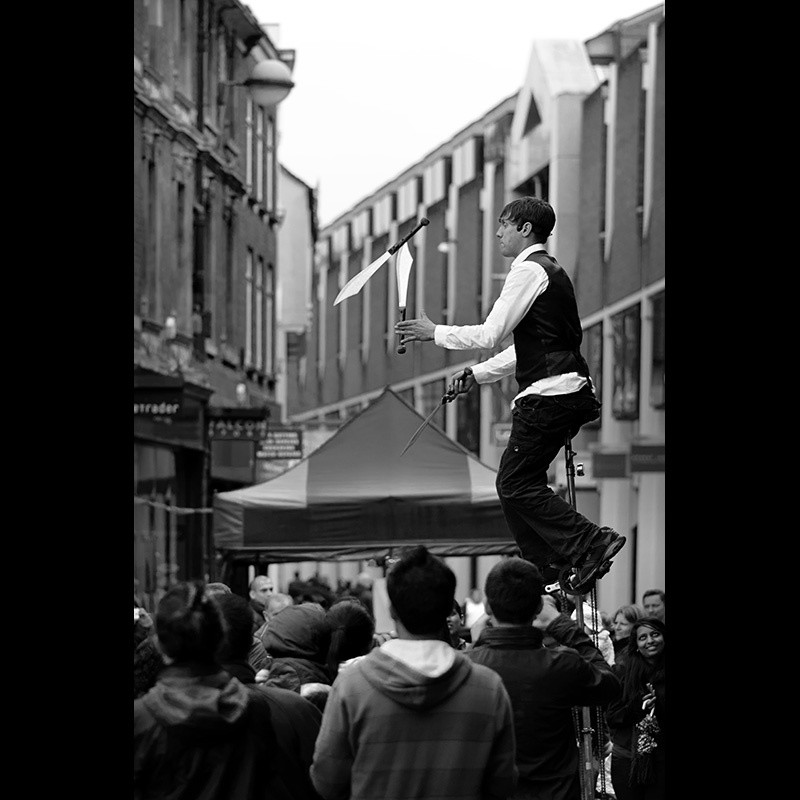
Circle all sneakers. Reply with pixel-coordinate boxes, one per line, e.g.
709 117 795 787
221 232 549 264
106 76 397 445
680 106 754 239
572 526 625 588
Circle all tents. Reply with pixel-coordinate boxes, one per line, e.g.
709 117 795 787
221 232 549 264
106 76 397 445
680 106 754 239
211 389 570 582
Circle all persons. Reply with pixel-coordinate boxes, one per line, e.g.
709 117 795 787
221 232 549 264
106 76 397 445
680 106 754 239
394 198 627 593
135 545 666 800
